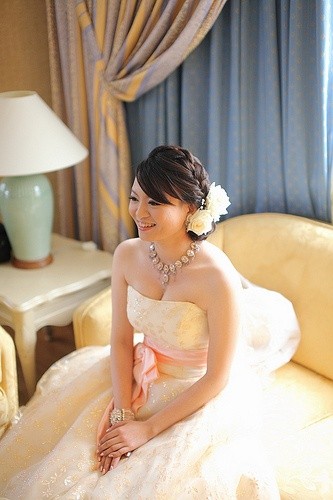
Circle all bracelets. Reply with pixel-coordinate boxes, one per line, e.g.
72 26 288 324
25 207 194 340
110 409 134 425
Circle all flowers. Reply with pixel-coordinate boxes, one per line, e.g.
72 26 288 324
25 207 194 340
187 182 231 236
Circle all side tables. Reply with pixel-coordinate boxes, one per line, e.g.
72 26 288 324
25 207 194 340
0 233 113 400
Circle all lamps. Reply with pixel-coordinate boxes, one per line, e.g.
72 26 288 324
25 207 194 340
0 90 88 270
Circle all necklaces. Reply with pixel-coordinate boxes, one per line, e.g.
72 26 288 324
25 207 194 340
148 237 199 284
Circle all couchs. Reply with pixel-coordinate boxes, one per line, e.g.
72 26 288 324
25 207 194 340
72 211 333 500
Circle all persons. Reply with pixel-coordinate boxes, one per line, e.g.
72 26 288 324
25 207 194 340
1 144 281 500
